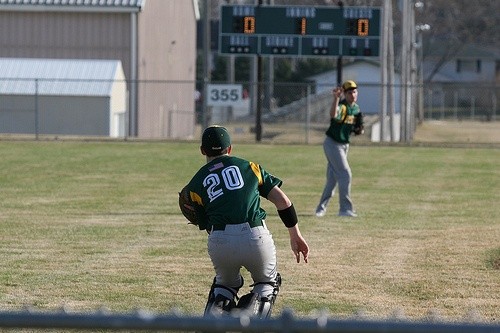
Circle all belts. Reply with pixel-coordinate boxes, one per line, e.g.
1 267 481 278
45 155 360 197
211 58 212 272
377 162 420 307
209 219 263 230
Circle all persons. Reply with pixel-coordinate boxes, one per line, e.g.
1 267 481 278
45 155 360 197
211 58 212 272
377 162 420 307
178 124 310 320
314 80 364 218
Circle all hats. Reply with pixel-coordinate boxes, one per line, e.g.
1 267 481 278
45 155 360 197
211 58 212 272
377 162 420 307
343 80 358 91
202 125 231 153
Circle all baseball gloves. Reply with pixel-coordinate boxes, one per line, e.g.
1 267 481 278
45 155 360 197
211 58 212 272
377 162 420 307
351 112 364 136
179 186 207 230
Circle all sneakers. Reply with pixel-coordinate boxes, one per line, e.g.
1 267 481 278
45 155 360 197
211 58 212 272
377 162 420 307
339 210 357 217
316 207 325 216
